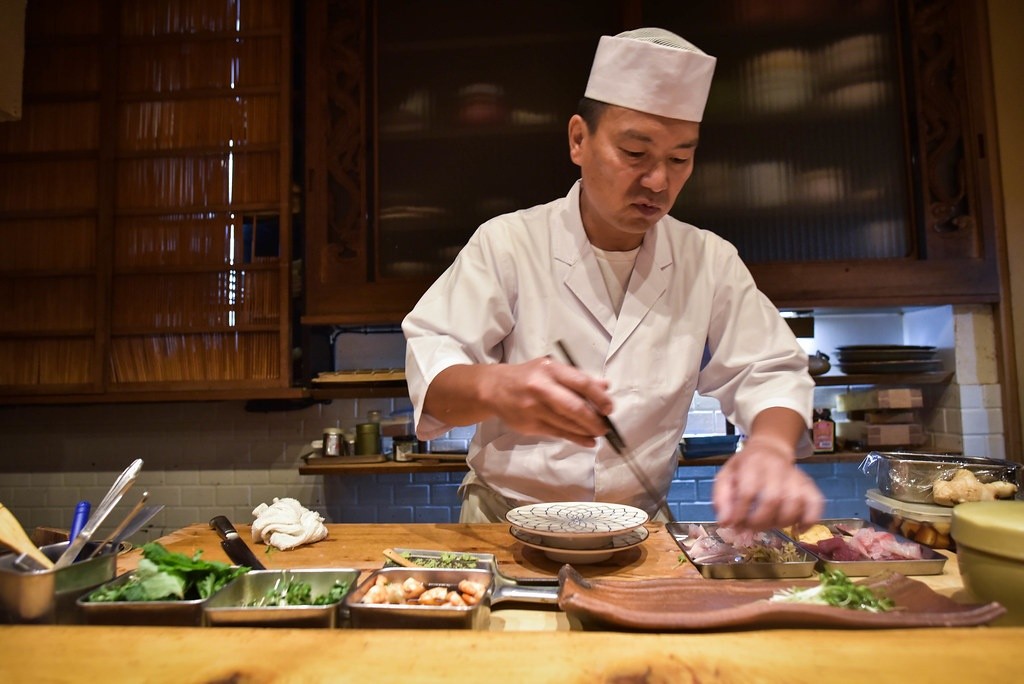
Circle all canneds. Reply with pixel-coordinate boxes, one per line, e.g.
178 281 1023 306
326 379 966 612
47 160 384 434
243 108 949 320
393 436 414 462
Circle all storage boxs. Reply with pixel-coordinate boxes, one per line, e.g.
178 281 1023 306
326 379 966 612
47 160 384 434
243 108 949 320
865 487 953 550
840 387 925 447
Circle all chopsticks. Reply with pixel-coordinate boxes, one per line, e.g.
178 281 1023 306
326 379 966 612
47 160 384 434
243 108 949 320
546 340 684 536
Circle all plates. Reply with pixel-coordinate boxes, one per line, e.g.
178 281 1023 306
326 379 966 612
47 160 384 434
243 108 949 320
831 344 943 374
509 525 649 563
685 159 884 210
557 564 1008 628
505 502 649 549
735 34 892 114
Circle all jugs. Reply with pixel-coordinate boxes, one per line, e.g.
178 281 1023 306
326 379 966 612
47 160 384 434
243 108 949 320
809 351 830 376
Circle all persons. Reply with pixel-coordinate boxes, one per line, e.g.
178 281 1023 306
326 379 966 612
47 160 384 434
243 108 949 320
398 26 823 534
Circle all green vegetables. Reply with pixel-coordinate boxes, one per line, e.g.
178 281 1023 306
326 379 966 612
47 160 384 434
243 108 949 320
255 579 347 604
88 544 250 600
770 570 894 612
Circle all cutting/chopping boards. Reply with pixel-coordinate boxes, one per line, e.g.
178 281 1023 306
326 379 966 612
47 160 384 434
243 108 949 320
116 521 705 579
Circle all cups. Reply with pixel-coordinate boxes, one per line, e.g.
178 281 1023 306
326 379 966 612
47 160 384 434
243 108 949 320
380 407 414 460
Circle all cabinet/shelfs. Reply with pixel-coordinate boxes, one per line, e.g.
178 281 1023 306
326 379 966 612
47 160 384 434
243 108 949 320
303 1 1000 323
297 364 959 474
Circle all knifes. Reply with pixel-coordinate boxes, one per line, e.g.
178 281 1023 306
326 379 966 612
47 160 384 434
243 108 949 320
393 546 559 587
208 515 267 570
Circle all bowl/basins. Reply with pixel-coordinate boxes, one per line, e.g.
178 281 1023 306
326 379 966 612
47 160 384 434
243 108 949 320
950 500 1024 622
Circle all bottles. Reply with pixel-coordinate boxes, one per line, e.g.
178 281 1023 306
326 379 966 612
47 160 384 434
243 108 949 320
323 409 379 456
812 407 836 454
392 435 428 462
